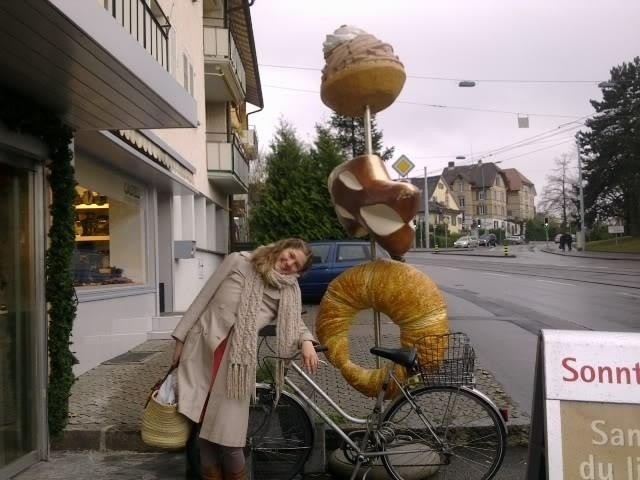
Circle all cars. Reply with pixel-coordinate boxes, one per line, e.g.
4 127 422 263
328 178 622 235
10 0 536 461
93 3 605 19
503 235 522 244
454 234 496 248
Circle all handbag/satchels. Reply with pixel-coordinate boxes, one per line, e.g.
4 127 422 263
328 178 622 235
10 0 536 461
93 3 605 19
141 365 191 450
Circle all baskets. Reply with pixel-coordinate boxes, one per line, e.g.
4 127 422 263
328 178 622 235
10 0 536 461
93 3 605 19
414 331 476 388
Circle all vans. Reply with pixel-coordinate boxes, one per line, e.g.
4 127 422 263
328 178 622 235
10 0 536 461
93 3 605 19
300 240 391 303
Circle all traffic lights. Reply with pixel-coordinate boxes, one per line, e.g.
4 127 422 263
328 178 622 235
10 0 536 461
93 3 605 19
544 218 549 230
478 219 480 228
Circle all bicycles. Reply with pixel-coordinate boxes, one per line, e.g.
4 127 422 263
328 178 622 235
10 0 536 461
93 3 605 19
192 312 509 480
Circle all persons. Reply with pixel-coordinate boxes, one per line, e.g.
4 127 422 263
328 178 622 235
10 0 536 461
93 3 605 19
171 237 320 480
559 234 572 251
